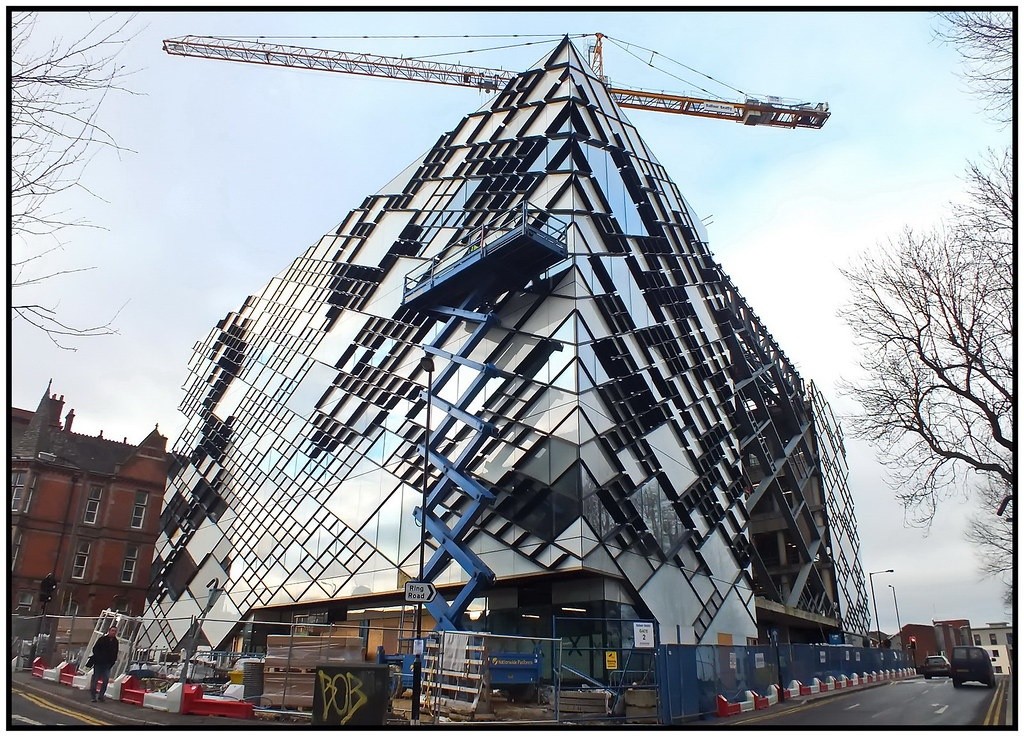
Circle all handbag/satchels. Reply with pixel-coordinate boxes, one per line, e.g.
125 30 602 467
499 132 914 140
86 656 94 668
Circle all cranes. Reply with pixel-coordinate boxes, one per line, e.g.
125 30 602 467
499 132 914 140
161 29 831 130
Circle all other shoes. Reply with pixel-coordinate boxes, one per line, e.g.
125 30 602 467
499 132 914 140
99 697 105 701
91 698 96 702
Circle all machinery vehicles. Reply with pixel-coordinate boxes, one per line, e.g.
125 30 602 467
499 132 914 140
374 201 570 698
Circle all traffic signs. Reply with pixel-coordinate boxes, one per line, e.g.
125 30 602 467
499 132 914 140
404 581 438 603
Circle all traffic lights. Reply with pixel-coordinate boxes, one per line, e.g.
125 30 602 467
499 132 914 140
910 636 917 650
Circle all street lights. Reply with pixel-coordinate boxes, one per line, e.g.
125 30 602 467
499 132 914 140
889 585 905 651
409 356 435 726
869 570 894 647
38 450 90 670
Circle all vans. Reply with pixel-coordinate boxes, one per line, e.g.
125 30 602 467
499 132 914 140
951 646 996 689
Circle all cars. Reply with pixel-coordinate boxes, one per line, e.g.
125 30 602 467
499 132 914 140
922 656 951 679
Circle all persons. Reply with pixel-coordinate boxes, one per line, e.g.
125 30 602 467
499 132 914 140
90 626 119 702
